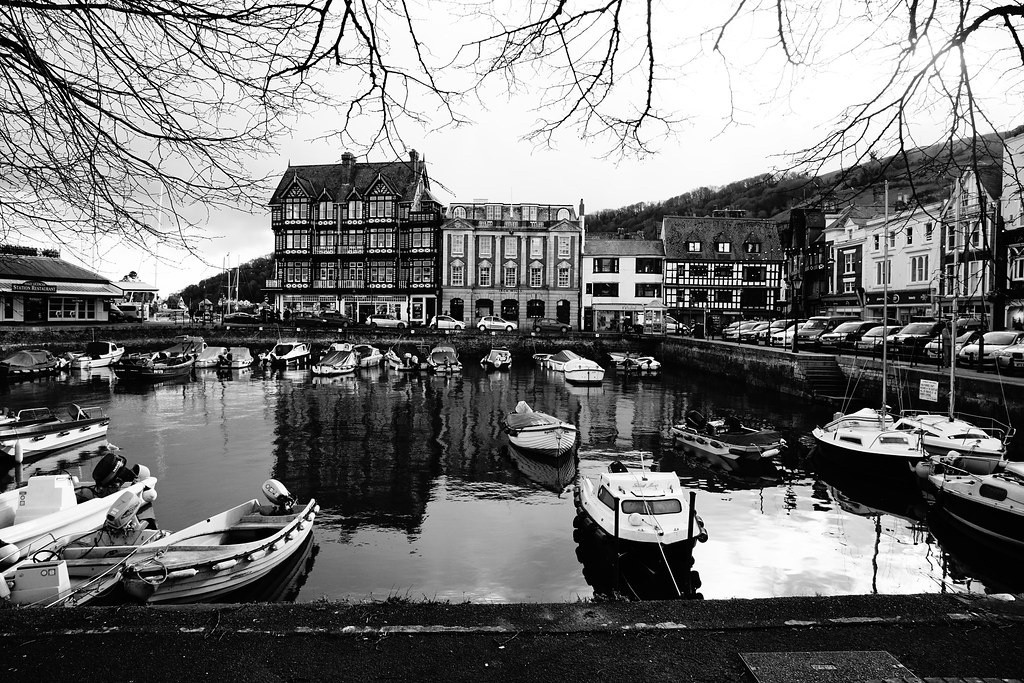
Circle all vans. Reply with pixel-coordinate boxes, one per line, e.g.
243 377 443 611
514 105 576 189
634 314 690 335
790 315 859 352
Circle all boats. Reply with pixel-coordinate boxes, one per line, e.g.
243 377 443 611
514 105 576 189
115 479 320 604
572 515 705 600
479 346 513 372
259 339 314 369
59 341 126 370
310 344 387 376
0 348 72 381
532 349 606 383
0 488 172 611
669 409 789 467
213 529 317 602
158 334 254 370
506 440 580 497
607 351 662 370
417 348 430 370
0 402 111 461
570 451 709 568
0 450 158 566
502 401 577 457
425 347 463 372
382 346 421 371
113 350 195 382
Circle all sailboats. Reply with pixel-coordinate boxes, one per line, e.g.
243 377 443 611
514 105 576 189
809 177 1024 555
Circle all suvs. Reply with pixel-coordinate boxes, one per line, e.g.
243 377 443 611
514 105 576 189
818 321 883 352
885 321 945 359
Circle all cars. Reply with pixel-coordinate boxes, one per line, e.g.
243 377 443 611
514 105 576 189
223 309 411 330
997 337 1024 375
721 317 806 348
532 318 573 334
924 328 986 364
430 314 467 331
960 330 1023 371
477 315 518 333
852 325 904 355
